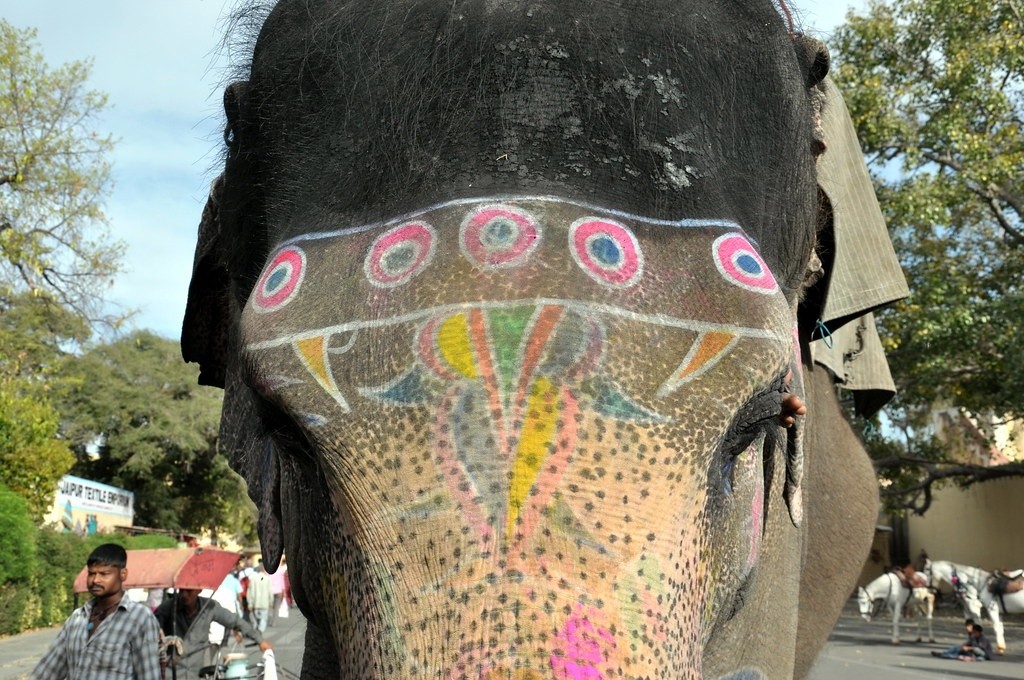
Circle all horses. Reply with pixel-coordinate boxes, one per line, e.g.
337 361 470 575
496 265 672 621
857 556 1024 656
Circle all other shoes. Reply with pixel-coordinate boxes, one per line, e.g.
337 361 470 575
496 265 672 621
931 650 943 657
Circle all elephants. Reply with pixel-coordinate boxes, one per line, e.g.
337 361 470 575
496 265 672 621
181 0 881 678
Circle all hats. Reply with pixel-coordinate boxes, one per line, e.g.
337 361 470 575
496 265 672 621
973 624 983 631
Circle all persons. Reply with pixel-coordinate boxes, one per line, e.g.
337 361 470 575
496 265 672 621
25 543 163 680
931 619 994 662
198 555 294 645
153 588 272 680
147 587 163 612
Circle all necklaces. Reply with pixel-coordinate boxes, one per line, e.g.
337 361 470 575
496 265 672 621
86 597 123 630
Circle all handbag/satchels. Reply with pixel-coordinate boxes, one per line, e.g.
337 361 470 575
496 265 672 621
278 597 288 618
